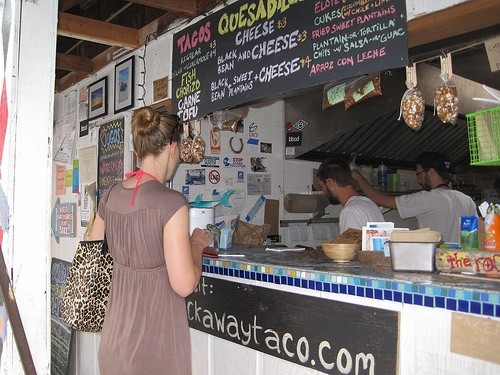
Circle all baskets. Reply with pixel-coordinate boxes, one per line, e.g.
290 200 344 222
465 106 500 166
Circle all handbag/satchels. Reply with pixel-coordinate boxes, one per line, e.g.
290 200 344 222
60 184 114 332
231 219 272 246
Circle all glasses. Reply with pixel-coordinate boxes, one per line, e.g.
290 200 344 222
169 115 180 145
416 171 424 177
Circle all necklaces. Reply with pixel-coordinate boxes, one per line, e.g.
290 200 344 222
435 183 449 189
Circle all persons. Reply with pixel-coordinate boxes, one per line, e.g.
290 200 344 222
88 106 215 375
352 152 478 243
318 157 386 236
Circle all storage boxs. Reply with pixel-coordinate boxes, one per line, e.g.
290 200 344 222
384 240 442 273
209 235 233 250
467 105 500 167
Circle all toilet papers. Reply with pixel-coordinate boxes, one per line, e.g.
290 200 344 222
284 192 317 213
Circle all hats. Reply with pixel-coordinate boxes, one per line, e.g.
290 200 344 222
416 151 455 182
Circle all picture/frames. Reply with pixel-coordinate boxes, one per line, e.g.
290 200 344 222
112 55 135 114
88 76 108 121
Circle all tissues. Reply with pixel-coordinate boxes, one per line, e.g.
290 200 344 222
458 201 489 251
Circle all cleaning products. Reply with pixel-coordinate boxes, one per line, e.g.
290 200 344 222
484 201 500 253
